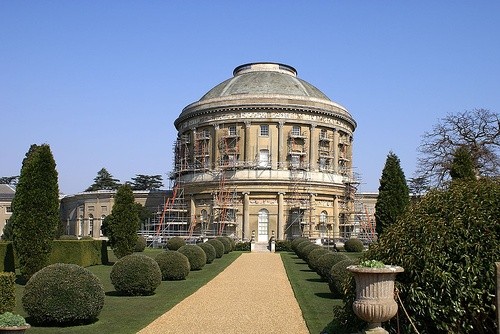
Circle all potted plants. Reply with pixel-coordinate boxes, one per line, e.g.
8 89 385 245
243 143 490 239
347 260 405 334
